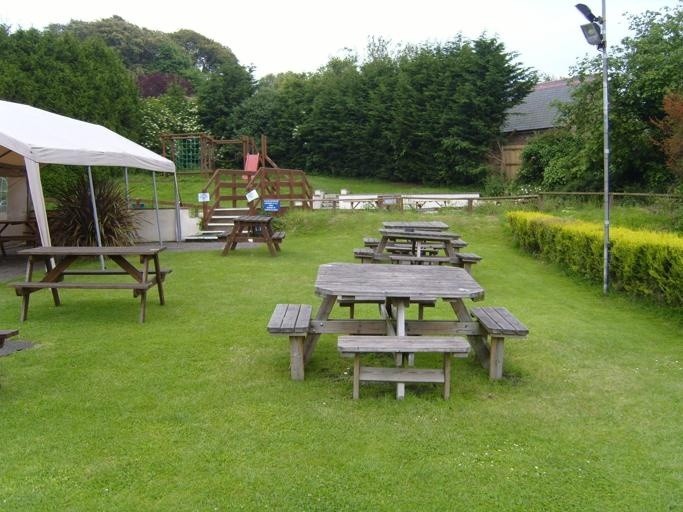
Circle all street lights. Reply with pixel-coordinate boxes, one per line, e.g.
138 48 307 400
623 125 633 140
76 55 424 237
573 0 611 298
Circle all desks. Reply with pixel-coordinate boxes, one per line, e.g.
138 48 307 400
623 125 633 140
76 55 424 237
0 220 38 257
223 216 276 258
314 261 484 400
15 245 167 321
380 219 449 253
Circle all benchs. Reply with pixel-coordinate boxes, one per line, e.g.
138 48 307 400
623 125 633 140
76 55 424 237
0 235 39 257
264 299 528 400
216 231 284 256
353 230 482 275
9 268 171 324
